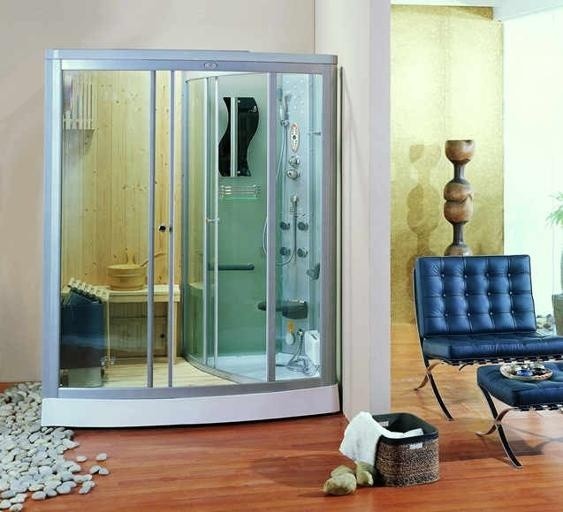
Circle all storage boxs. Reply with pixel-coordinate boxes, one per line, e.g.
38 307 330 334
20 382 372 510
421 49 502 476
365 411 441 487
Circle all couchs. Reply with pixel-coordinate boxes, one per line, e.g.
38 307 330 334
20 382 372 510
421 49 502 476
414 255 563 421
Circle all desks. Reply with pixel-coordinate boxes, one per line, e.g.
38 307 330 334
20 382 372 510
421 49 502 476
62 283 180 365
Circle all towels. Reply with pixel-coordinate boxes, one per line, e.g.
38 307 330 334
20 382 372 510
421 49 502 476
339 410 424 468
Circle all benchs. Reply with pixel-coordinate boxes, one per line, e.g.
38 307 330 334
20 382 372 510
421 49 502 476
476 364 563 468
